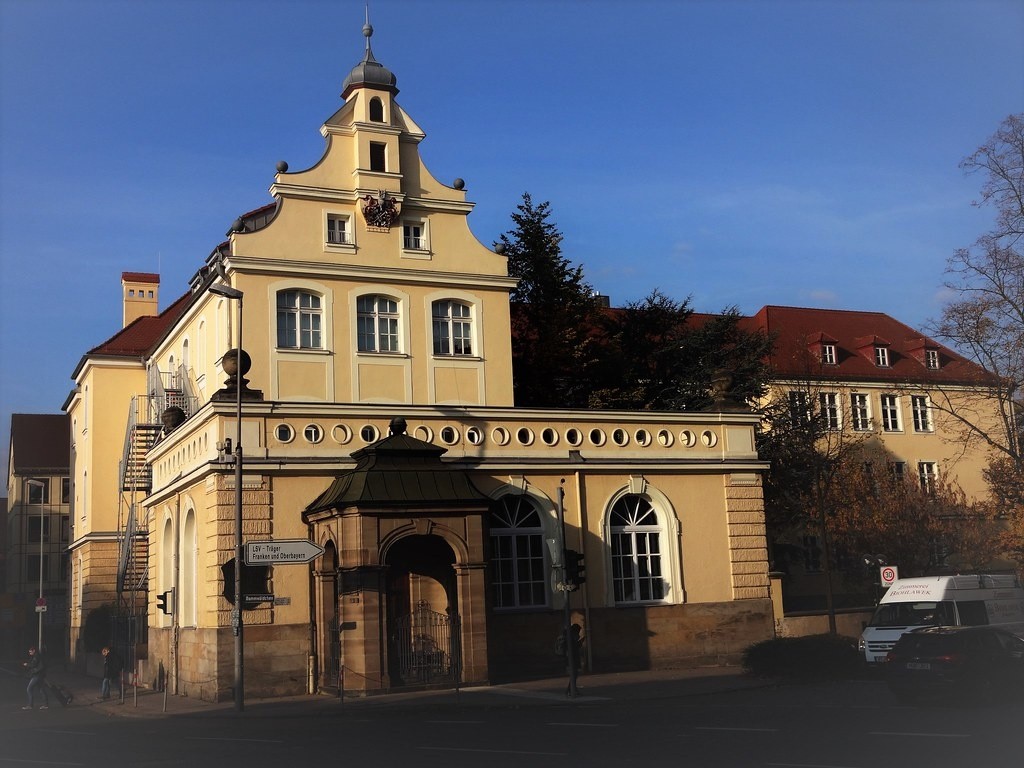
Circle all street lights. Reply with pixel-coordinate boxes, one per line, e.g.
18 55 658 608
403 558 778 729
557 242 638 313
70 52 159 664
207 282 244 712
27 480 44 656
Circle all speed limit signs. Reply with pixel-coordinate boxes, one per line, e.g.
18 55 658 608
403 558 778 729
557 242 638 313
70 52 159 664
880 566 898 588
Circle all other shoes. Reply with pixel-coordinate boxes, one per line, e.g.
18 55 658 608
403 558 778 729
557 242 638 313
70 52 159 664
96 695 110 699
22 706 33 710
39 705 48 710
119 688 126 699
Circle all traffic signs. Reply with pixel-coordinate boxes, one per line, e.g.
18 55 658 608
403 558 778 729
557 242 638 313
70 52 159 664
244 538 326 566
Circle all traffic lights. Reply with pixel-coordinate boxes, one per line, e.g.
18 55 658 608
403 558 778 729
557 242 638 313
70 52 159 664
566 549 585 593
157 592 167 613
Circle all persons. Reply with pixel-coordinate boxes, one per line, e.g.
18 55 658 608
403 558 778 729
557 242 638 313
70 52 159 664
21 647 49 709
566 624 586 695
96 647 126 700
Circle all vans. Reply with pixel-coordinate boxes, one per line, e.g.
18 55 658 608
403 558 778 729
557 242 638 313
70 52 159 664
857 572 1024 667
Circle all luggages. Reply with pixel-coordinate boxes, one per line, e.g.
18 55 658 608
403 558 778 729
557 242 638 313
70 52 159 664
43 678 73 705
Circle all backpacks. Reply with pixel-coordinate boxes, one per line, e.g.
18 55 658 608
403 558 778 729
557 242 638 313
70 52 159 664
554 625 577 658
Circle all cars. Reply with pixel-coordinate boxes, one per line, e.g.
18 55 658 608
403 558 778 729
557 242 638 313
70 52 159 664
885 624 1024 706
